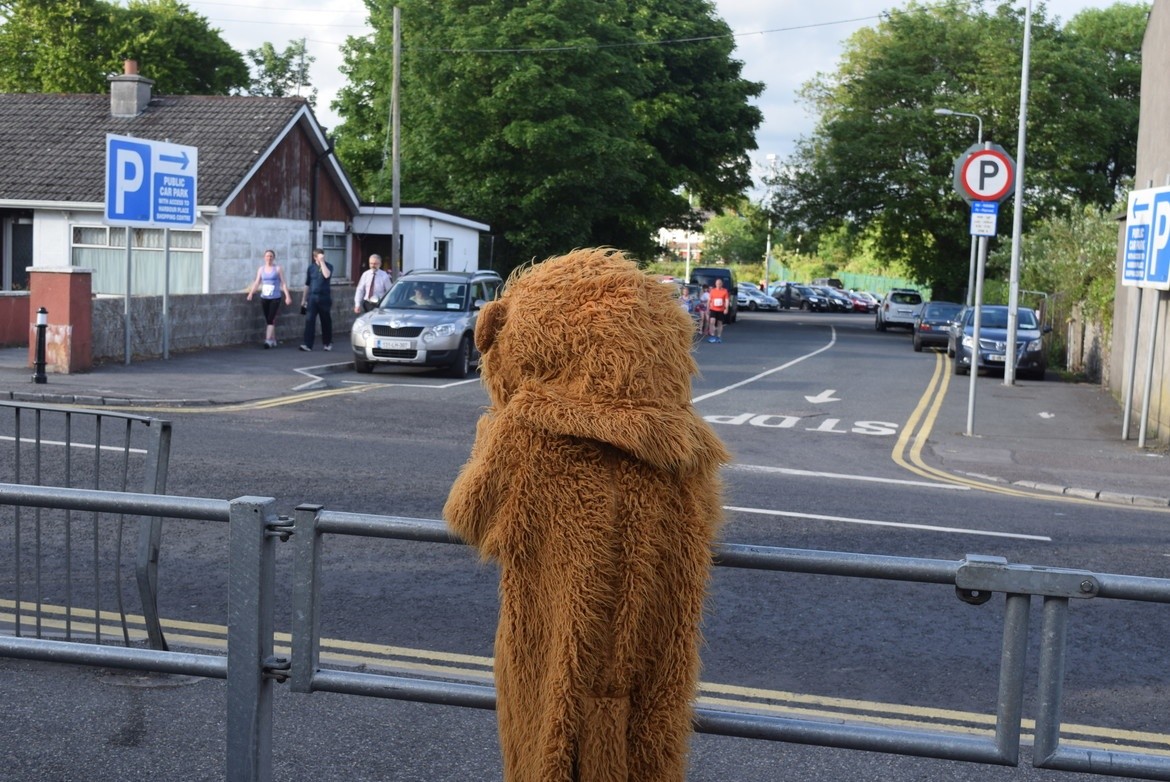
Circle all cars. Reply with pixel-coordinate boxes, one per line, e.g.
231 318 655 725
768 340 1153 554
736 283 780 312
912 302 963 352
953 303 1053 379
758 280 882 313
676 284 707 334
945 306 972 358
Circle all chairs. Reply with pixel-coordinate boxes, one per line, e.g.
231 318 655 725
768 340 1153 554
457 285 465 298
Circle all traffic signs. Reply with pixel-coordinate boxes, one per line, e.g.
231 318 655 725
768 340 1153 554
1121 184 1170 292
103 132 199 228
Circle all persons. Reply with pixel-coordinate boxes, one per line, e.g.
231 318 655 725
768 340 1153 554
759 284 764 292
784 283 792 310
297 250 333 352
679 278 730 343
246 250 292 348
354 254 393 314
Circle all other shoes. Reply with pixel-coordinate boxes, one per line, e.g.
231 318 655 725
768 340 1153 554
264 339 277 348
717 337 722 343
300 345 313 352
324 343 331 350
708 336 715 343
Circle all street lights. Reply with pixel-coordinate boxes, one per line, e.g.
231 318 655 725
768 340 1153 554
933 107 984 440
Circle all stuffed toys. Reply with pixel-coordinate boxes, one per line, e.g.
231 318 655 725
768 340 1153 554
444 249 726 782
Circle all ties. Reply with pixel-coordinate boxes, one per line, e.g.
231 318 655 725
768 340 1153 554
367 273 377 300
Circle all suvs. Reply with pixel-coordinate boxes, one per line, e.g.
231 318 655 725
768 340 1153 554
874 288 926 332
350 267 505 379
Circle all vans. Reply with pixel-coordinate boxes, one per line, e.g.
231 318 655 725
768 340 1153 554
689 267 739 324
